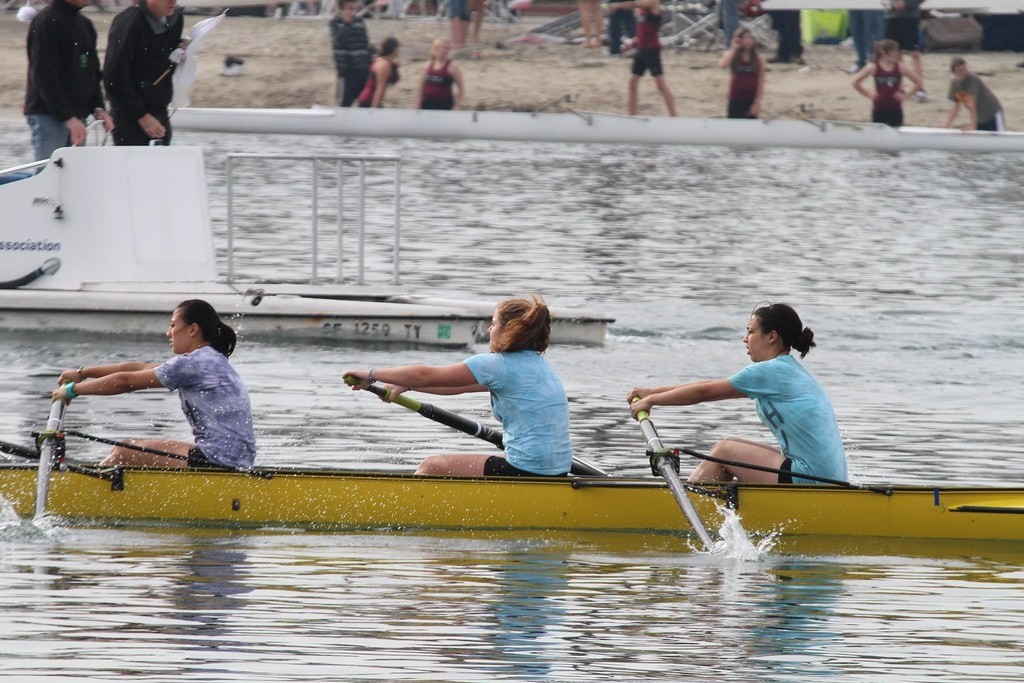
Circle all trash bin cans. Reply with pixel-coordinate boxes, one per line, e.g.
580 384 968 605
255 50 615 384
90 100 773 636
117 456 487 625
798 8 851 45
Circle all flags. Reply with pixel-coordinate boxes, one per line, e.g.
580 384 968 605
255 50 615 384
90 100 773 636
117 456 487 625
172 12 227 107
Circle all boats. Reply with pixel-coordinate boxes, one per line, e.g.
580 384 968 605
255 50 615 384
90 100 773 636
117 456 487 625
173 106 1023 152
0 120 616 347
0 373 1024 541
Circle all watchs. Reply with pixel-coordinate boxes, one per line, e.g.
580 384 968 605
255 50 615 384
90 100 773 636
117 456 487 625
66 382 78 398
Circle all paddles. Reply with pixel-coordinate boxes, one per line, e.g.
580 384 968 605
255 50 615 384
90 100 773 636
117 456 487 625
631 395 715 553
343 374 610 477
34 378 71 518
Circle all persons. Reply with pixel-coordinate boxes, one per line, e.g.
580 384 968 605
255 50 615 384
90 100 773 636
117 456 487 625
342 298 572 477
23 0 114 171
578 0 637 55
716 0 806 65
626 301 847 483
851 40 924 126
417 37 464 109
719 27 765 120
330 0 379 107
103 0 188 146
355 37 401 108
849 0 926 92
448 0 487 47
601 0 677 116
51 298 257 468
944 56 1006 133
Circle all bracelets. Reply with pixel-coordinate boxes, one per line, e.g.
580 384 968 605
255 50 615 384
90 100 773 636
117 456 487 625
368 369 377 384
77 366 87 380
